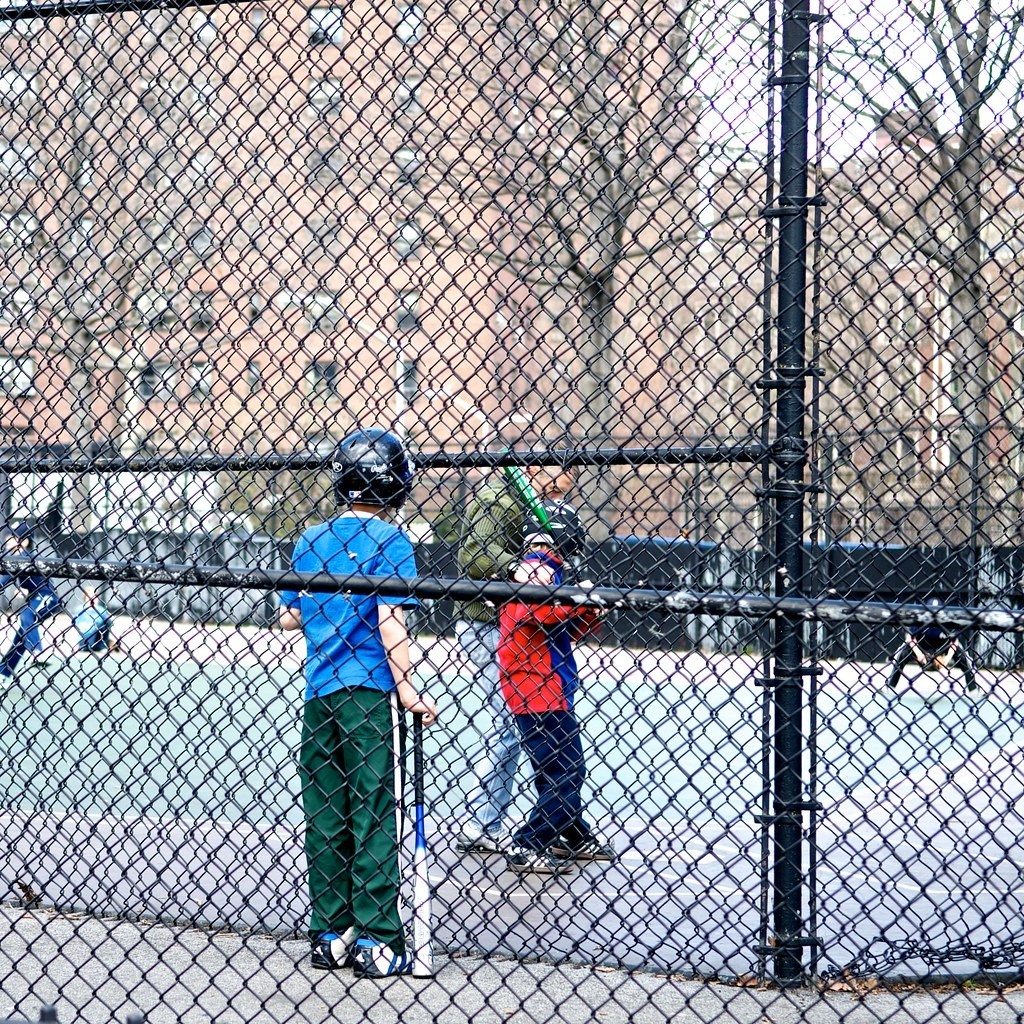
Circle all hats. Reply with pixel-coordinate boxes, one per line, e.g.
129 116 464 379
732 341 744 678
3 520 33 541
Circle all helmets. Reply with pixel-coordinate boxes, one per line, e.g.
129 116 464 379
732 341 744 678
522 500 586 556
334 429 415 509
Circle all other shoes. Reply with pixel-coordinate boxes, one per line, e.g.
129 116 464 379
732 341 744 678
31 655 52 669
0 673 8 690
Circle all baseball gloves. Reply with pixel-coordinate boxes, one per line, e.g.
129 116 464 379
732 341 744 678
923 651 944 672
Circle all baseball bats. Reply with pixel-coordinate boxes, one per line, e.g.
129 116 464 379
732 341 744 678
496 445 609 619
411 710 437 980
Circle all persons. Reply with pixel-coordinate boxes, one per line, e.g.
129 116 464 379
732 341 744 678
0 520 58 690
451 440 617 874
73 587 121 652
883 598 979 696
278 429 439 979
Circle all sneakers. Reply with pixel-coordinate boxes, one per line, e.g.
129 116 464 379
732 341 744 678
353 939 417 978
549 834 617 861
506 846 574 873
589 833 616 852
311 926 363 969
456 821 515 853
